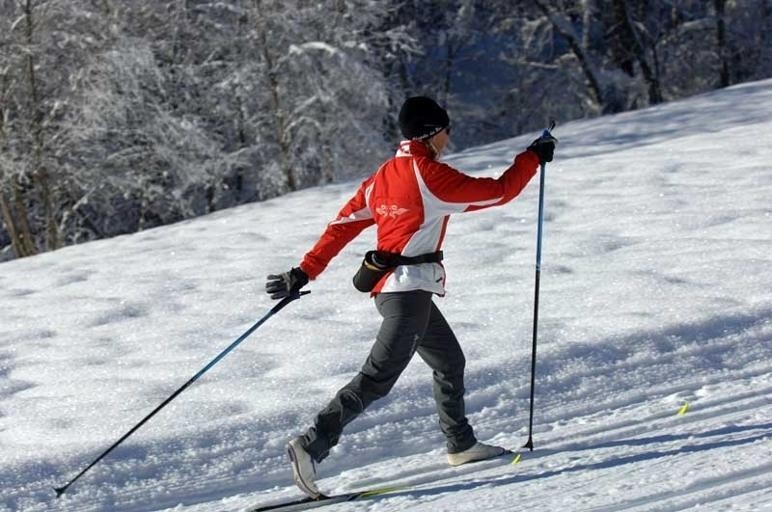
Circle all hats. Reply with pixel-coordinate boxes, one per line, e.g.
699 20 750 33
399 95 450 140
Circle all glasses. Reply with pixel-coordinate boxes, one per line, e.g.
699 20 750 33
444 126 452 136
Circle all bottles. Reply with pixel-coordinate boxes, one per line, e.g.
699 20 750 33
352 249 388 292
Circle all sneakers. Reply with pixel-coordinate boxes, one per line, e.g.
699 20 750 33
284 438 321 500
448 442 504 466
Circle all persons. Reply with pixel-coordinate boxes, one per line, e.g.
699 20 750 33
266 95 557 501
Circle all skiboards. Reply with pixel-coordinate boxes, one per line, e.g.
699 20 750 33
244 404 689 512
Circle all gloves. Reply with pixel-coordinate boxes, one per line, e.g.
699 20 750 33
265 267 309 300
526 128 559 166
